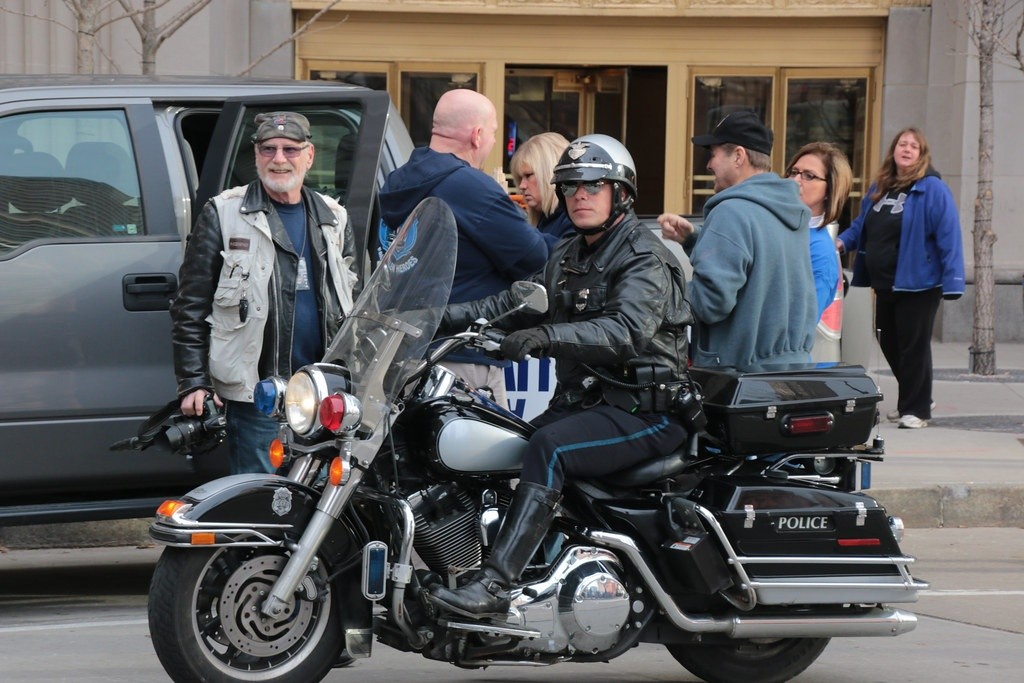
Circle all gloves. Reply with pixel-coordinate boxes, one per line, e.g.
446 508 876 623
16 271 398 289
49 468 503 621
501 328 550 364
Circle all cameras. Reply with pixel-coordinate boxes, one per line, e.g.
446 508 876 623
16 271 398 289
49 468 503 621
164 400 225 451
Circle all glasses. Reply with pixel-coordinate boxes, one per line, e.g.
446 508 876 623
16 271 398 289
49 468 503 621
787 168 826 182
257 141 311 158
559 178 605 196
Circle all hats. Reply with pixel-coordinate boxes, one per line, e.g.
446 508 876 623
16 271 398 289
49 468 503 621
250 111 312 142
690 110 773 155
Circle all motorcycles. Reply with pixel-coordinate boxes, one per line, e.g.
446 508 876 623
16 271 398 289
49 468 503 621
147 197 929 683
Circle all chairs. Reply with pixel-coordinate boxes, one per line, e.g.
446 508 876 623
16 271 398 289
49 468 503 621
0 142 141 198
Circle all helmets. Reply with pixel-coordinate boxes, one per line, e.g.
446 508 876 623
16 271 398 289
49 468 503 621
550 133 638 201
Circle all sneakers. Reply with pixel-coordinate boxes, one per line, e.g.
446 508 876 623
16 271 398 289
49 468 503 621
886 397 936 419
897 414 929 428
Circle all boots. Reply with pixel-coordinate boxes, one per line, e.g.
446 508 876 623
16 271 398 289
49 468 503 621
425 481 561 619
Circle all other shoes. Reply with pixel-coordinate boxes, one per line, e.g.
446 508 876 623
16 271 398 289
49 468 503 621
332 648 356 667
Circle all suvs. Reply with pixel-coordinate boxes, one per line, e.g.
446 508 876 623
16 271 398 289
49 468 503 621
0 72 417 570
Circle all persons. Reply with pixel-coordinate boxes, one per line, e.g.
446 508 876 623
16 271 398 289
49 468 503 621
833 126 966 428
509 132 575 245
368 87 549 413
656 110 818 467
783 142 854 329
355 134 694 623
169 111 368 475
